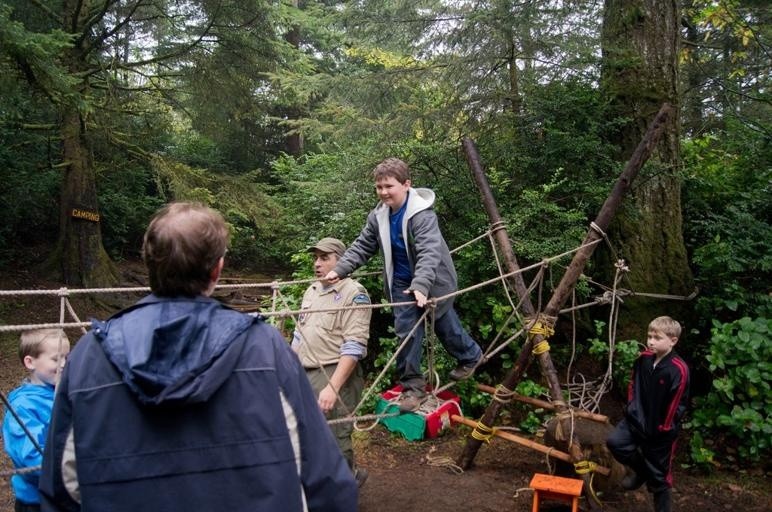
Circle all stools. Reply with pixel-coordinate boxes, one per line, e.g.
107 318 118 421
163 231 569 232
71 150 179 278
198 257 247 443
529 472 585 512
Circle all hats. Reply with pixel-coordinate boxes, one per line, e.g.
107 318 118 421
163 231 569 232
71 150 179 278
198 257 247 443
307 237 346 257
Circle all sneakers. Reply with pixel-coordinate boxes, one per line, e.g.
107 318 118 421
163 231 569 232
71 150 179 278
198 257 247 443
622 467 646 490
400 388 428 412
448 350 484 380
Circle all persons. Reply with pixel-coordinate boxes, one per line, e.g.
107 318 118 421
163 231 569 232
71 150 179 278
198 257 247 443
605 315 690 511
35 199 359 511
0 326 73 512
319 154 483 413
283 234 372 469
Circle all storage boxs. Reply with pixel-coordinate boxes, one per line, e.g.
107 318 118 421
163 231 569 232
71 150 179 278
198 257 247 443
374 383 461 442
